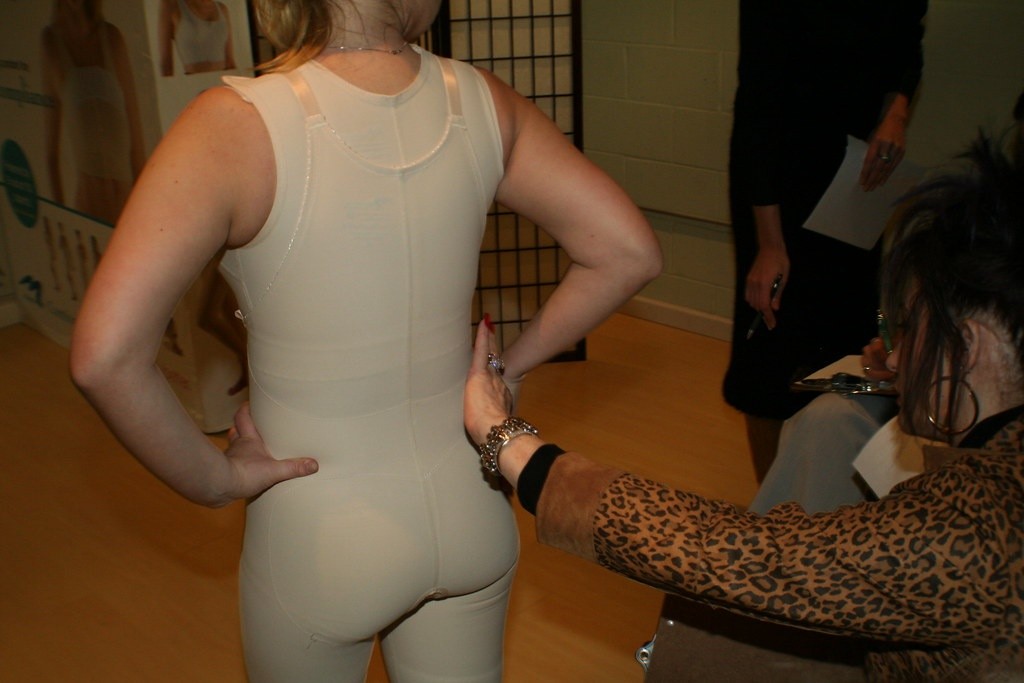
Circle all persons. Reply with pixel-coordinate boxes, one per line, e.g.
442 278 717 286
157 0 238 77
195 251 250 396
38 0 149 227
714 0 928 421
41 216 104 300
460 138 1024 683
70 0 664 683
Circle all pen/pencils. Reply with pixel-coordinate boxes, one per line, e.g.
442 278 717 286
876 308 893 356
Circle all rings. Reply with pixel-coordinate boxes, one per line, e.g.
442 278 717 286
877 153 889 160
487 352 505 376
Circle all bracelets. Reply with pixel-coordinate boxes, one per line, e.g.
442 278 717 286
479 417 540 479
502 374 526 382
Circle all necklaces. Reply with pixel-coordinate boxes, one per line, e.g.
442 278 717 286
328 38 408 55
